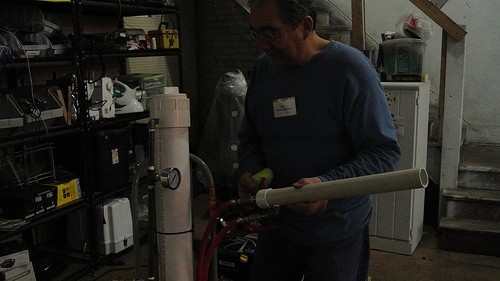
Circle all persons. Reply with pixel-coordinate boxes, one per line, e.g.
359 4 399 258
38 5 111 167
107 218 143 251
236 1 401 281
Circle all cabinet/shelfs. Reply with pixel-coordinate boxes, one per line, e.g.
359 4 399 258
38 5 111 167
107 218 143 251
0 0 98 281
76 0 186 281
